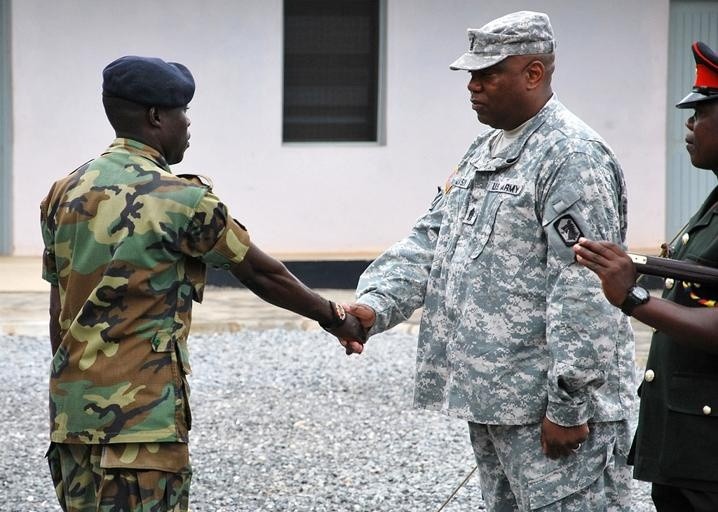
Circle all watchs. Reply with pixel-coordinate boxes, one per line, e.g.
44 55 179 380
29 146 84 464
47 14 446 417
619 284 652 316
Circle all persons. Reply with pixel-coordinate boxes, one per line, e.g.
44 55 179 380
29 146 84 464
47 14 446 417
333 10 649 512
570 42 717 512
39 55 369 510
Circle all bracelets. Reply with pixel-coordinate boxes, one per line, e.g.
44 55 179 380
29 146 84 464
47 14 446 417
319 299 348 333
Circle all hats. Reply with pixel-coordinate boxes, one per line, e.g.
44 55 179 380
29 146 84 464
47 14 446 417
102 56 196 108
674 40 718 109
449 10 556 72
